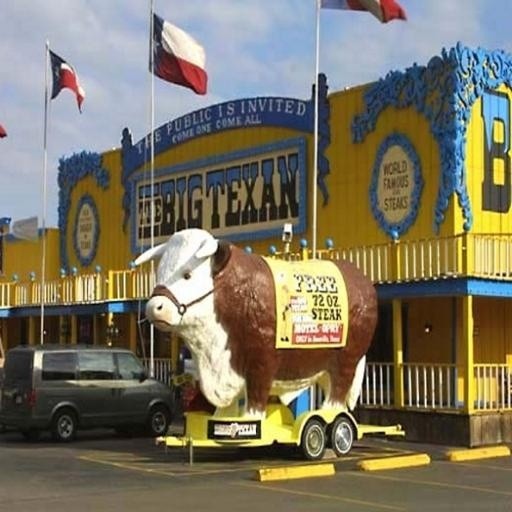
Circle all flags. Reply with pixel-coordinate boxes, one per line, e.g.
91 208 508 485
50 48 87 114
150 14 208 95
320 0 408 24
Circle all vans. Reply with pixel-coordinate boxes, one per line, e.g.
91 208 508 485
0 344 177 441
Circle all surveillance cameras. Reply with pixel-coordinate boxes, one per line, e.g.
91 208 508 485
281 222 294 243
